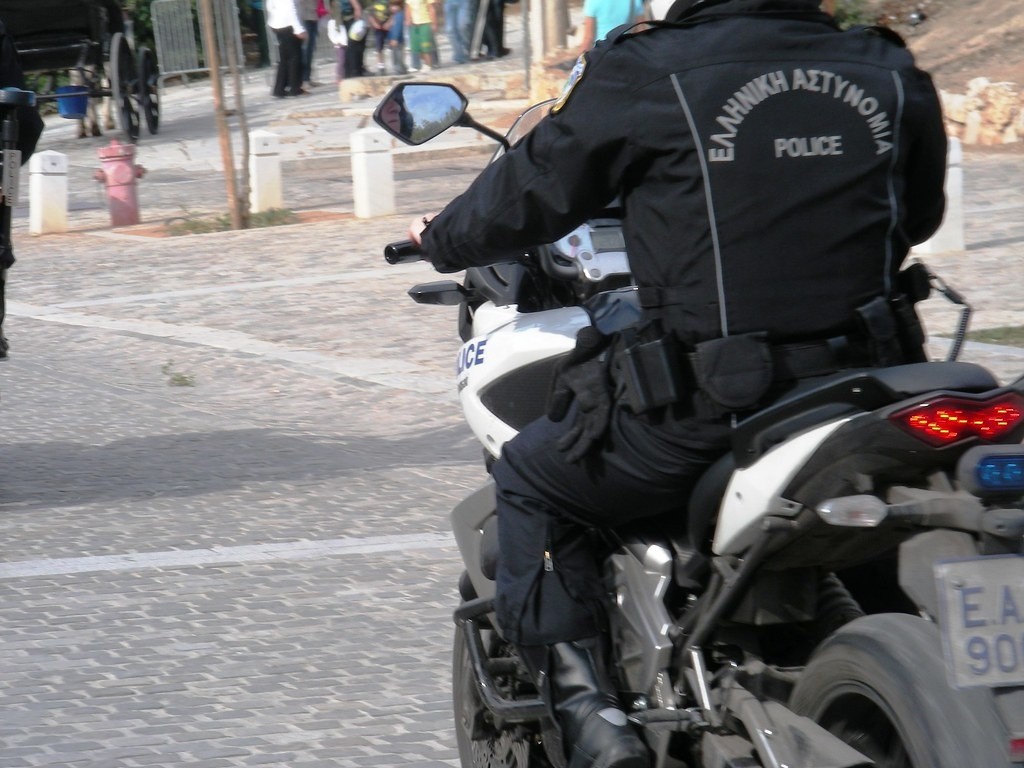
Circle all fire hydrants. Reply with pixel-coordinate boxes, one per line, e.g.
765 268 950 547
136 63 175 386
93 139 148 227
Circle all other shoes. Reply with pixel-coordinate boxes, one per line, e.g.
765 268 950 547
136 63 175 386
273 86 312 98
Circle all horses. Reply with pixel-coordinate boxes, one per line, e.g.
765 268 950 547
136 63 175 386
71 63 117 139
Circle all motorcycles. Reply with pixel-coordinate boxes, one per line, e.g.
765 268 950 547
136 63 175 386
373 81 1024 768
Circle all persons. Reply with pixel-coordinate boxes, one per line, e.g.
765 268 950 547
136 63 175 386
560 1 649 60
379 85 413 138
249 0 511 96
0 28 45 362
405 1 950 767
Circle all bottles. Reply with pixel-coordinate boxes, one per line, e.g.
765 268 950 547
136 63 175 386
349 18 369 41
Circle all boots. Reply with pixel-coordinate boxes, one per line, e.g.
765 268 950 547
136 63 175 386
518 630 653 768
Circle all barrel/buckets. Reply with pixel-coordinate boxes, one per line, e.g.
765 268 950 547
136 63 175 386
56 85 89 120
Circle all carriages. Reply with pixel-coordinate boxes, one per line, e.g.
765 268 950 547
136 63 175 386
0 0 161 143
